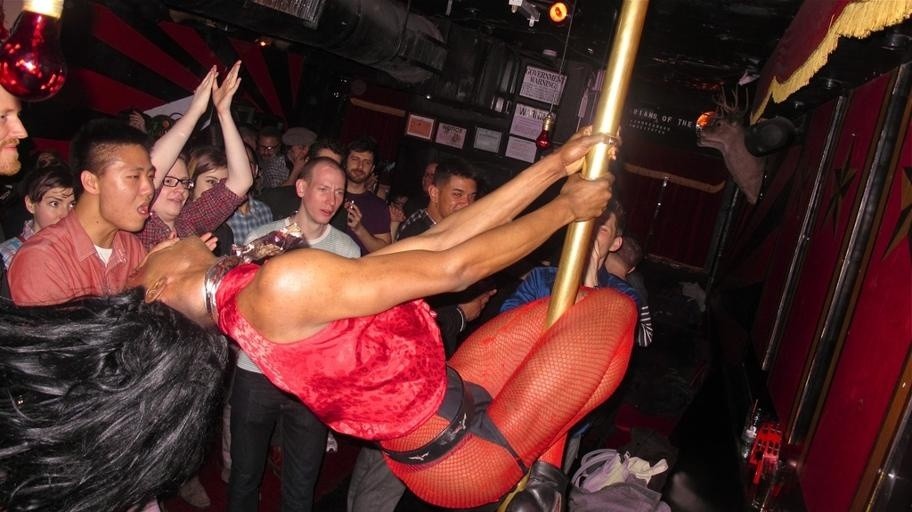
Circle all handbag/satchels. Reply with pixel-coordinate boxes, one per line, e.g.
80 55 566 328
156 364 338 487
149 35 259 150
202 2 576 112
571 449 668 493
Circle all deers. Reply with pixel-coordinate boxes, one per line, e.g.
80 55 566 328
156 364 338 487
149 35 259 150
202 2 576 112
691 79 766 205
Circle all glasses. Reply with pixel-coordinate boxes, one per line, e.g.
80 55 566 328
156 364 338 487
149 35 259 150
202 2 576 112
259 145 277 152
162 175 195 189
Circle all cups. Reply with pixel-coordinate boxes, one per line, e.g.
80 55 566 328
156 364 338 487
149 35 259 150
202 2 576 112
750 456 796 512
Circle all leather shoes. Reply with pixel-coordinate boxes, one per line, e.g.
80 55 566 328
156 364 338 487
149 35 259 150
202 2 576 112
179 475 211 508
508 462 570 512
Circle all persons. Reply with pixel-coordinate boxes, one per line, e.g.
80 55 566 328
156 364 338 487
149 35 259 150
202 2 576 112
0 58 655 512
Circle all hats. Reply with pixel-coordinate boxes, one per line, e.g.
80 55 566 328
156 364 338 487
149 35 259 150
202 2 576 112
282 127 318 147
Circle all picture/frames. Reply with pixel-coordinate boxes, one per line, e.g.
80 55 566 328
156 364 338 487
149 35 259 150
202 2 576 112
402 63 570 165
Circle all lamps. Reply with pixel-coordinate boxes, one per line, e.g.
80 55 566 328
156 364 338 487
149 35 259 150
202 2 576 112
535 0 577 151
0 1 77 104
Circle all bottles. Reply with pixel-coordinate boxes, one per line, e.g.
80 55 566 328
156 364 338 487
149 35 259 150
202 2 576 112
738 406 770 462
